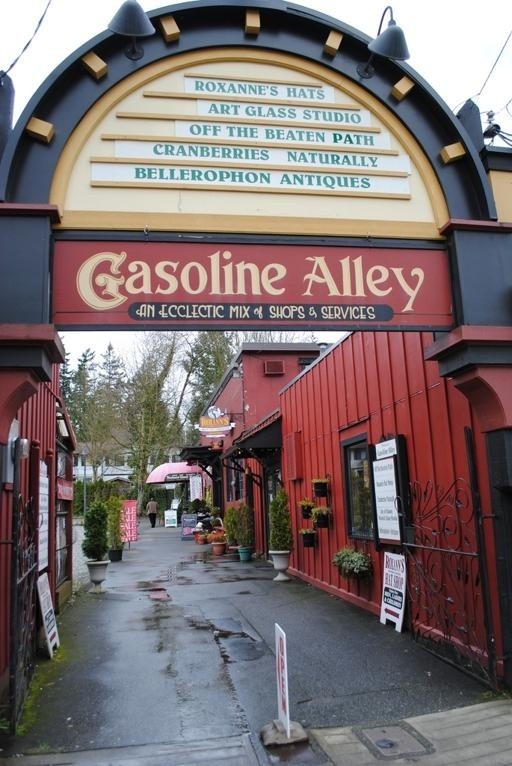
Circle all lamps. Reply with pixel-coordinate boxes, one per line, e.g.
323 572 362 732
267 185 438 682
106 0 157 62
356 1 411 81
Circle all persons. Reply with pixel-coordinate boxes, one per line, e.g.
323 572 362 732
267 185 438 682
145 497 159 528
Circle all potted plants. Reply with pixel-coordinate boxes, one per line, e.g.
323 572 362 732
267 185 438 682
80 494 115 596
183 473 375 584
104 496 128 565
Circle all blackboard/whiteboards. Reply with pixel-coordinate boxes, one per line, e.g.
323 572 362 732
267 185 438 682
181 514 198 538
